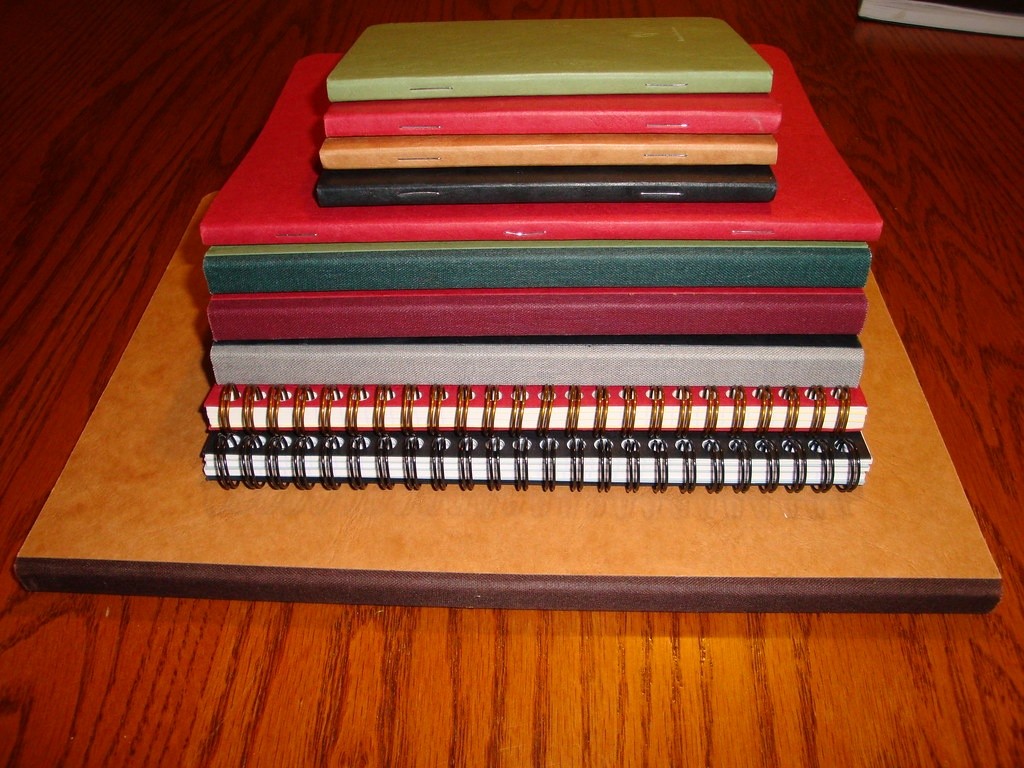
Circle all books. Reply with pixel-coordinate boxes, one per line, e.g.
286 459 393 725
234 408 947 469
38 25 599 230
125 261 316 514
203 383 869 433
210 332 866 390
859 0 1024 38
326 17 773 101
199 434 873 491
203 244 873 295
200 43 884 246
207 292 869 343
13 192 1003 611
